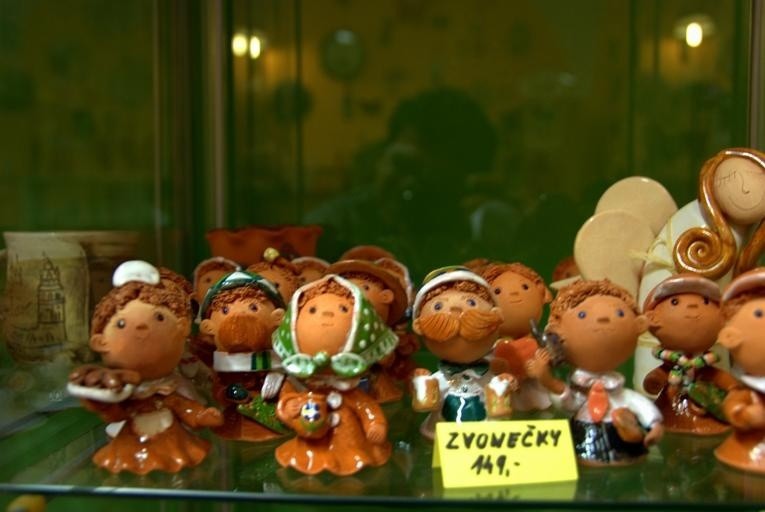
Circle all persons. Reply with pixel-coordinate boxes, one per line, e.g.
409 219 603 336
570 145 765 398
242 71 360 218
305 82 545 244
59 242 764 482
58 242 765 482
